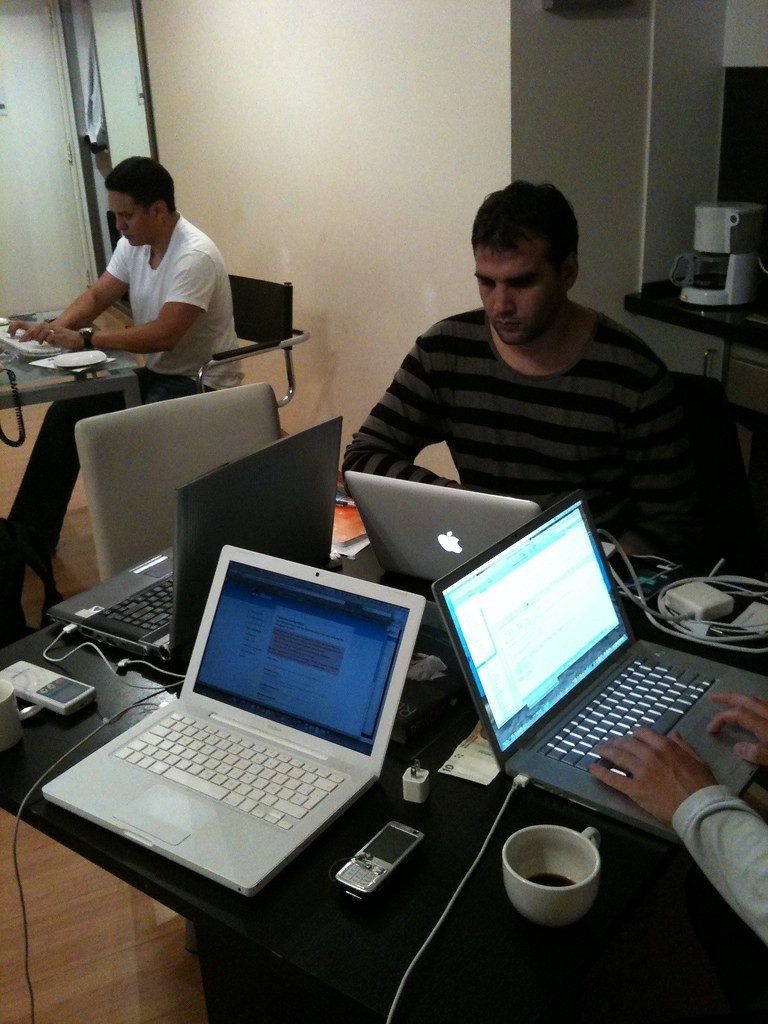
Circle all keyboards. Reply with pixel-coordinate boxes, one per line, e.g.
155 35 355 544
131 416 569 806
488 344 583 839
0 322 60 364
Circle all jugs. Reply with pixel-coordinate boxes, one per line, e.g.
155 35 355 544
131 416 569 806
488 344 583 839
672 253 728 289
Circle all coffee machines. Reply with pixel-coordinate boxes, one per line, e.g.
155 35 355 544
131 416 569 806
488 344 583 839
680 201 766 305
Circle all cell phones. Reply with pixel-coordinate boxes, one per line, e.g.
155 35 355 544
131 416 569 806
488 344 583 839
616 565 671 601
334 820 425 898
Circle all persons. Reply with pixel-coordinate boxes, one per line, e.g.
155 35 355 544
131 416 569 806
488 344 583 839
6 157 246 558
590 690 768 945
342 179 710 561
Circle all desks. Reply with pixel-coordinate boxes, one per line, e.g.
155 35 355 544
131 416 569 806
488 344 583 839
0 308 142 408
1 543 768 1024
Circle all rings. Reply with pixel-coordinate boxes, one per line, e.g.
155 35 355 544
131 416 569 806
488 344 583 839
49 329 53 334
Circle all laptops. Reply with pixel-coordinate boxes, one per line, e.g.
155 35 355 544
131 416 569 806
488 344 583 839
40 413 768 899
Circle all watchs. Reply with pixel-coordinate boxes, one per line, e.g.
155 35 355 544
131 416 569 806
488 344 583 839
79 327 94 348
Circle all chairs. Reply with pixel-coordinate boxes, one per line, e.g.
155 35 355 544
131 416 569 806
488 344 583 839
673 369 768 579
196 273 309 407
71 385 281 582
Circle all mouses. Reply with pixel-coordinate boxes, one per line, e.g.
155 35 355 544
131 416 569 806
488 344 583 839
0 317 11 325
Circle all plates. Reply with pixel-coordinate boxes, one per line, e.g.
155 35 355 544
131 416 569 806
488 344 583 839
54 350 106 368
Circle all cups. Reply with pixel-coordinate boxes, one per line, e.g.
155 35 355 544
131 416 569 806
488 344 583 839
502 826 601 924
0 680 24 751
